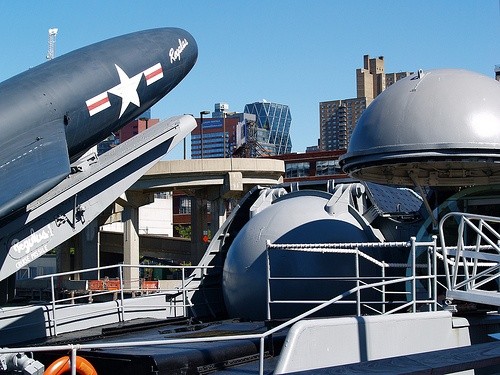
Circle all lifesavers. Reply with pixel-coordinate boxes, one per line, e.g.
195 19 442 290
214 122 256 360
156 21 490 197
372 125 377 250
41 355 98 375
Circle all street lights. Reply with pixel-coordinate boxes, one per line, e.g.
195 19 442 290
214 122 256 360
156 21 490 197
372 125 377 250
228 134 235 157
200 111 210 158
139 117 148 129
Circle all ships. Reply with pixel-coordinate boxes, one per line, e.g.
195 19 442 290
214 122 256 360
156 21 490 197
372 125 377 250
0 25 500 375
